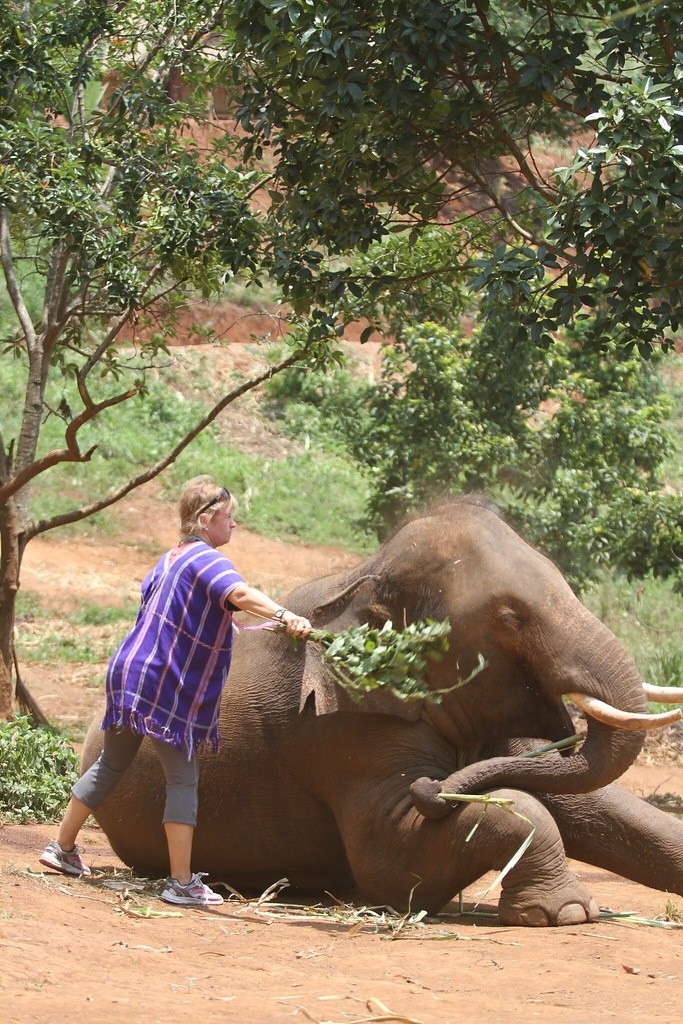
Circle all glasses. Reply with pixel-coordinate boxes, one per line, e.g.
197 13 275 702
196 487 230 521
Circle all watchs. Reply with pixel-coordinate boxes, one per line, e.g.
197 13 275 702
275 608 290 619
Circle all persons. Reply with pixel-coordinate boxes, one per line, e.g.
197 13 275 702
38 480 311 904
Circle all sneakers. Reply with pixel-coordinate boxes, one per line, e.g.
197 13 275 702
39 839 92 876
160 872 223 905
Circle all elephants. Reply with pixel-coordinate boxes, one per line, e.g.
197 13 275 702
79 493 682 928
